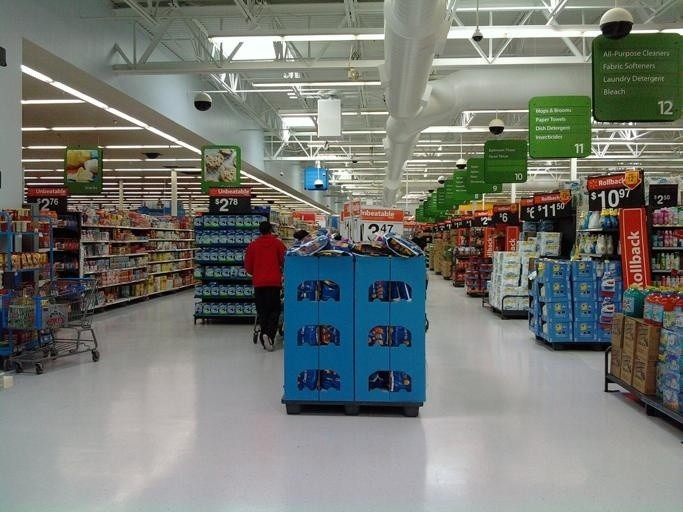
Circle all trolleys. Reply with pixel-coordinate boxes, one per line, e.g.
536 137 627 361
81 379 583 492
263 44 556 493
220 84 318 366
245 269 286 355
7 275 101 375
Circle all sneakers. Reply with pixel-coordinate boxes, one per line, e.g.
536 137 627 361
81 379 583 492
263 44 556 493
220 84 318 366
263 335 272 351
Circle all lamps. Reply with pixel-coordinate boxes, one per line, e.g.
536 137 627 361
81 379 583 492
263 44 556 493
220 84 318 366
313 162 324 191
468 1 486 44
437 134 466 185
600 2 633 41
487 111 506 136
192 73 213 111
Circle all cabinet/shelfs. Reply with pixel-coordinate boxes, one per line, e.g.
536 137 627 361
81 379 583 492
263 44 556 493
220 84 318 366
577 207 682 288
281 233 428 420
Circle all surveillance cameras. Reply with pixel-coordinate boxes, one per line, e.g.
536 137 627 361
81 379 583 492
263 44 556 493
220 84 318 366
438 175 446 185
352 155 358 163
598 7 635 40
419 197 424 202
193 92 212 112
455 157 467 170
426 187 434 194
487 119 505 136
472 30 484 42
313 180 323 190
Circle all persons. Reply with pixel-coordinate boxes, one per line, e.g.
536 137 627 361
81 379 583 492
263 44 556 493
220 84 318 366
244 221 287 353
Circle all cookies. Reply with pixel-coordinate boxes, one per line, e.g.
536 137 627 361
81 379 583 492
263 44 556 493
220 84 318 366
205 147 236 183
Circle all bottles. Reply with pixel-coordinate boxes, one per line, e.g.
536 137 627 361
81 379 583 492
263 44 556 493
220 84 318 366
601 298 614 323
53 221 78 270
623 206 683 333
1 208 50 233
81 210 193 306
577 208 622 255
601 260 615 292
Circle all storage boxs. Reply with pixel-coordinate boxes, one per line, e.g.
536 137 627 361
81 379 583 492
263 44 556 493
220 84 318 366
486 232 622 343
609 312 660 395
